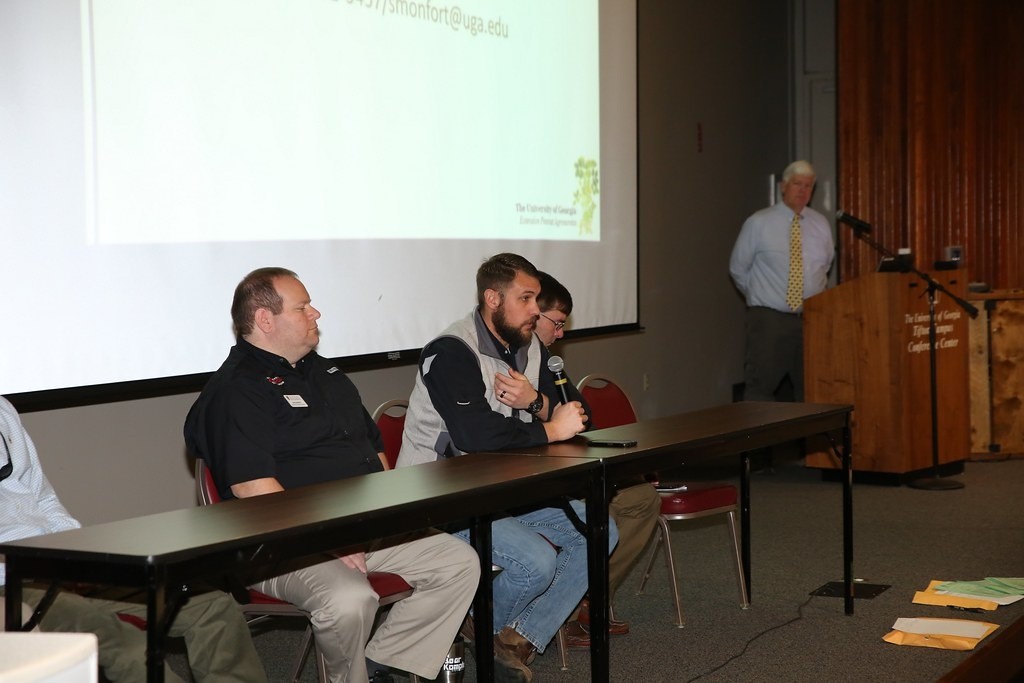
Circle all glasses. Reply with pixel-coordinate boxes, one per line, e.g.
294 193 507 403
540 312 566 331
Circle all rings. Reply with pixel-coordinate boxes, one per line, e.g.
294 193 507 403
500 390 506 398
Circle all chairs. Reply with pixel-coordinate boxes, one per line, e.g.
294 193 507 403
196 458 419 683
372 398 569 670
577 373 749 629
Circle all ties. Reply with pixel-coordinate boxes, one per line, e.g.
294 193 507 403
786 214 804 313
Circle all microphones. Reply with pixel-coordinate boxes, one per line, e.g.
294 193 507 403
548 356 572 405
836 209 871 232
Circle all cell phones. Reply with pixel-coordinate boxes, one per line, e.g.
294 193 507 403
587 440 637 447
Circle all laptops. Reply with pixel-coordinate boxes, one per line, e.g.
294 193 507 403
876 254 916 273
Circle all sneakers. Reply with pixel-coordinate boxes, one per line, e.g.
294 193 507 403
459 613 536 666
576 603 630 637
469 626 535 683
555 620 590 651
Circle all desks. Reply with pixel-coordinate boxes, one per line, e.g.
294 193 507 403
0 453 616 683
502 400 854 683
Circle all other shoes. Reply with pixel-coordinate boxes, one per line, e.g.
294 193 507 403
368 670 395 683
750 463 764 475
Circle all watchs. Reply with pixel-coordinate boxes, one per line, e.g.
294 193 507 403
525 389 543 414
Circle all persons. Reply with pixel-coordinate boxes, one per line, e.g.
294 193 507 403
727 159 839 455
395 252 663 678
0 392 269 683
185 266 483 683
